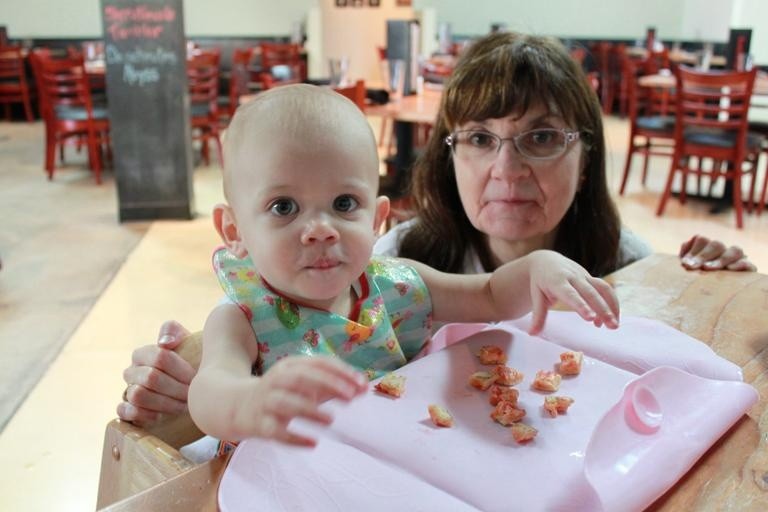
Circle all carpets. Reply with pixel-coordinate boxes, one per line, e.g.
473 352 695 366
0 110 207 436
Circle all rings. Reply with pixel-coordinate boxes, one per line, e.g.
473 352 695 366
121 385 128 402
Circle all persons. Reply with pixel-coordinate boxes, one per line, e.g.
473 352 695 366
117 32 759 427
634 26 665 56
187 79 624 468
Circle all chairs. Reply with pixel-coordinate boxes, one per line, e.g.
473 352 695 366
28 45 250 187
1 24 306 158
619 57 766 231
333 41 589 229
592 27 746 119
97 331 241 512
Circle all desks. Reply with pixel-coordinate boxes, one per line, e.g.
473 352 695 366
98 242 768 512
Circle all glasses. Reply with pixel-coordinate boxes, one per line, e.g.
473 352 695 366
443 128 580 162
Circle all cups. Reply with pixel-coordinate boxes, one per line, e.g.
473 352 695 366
659 70 669 77
86 42 105 65
378 59 406 100
